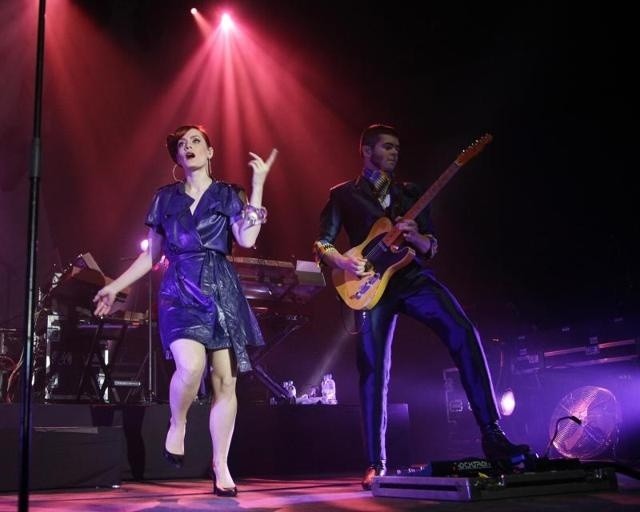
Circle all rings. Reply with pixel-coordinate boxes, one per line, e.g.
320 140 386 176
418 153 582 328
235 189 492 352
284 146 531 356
106 303 112 306
352 264 357 270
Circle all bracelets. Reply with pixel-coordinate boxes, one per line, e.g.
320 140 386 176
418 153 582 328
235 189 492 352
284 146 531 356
240 200 267 230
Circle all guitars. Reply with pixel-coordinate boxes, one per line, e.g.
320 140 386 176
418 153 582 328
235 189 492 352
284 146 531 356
332 131 493 312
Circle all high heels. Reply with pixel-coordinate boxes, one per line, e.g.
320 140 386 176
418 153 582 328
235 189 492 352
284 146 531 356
160 422 188 473
209 466 238 497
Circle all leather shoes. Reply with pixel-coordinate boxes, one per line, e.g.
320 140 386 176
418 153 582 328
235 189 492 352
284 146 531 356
362 463 387 491
481 431 531 466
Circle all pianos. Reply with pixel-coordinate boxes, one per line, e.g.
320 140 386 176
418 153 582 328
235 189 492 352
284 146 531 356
227 255 327 304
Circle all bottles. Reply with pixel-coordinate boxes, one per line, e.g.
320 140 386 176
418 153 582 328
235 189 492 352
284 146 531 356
282 379 298 400
321 373 338 405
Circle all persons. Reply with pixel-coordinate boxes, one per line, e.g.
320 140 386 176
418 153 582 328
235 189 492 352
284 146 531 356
312 123 532 490
92 125 278 496
